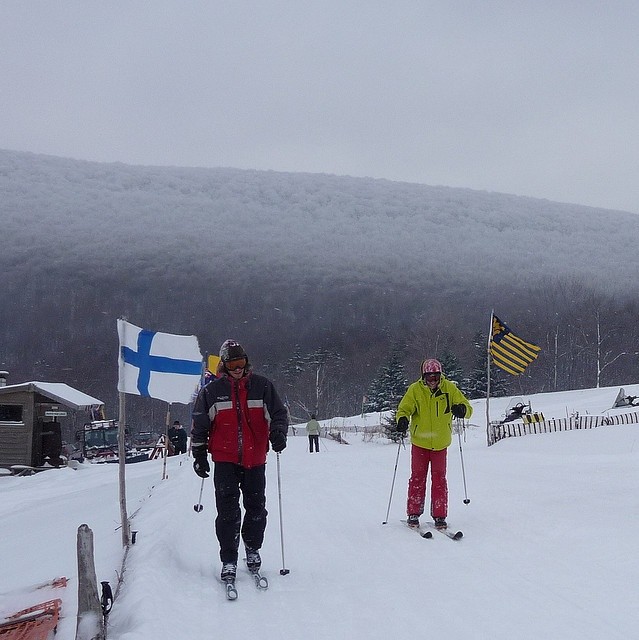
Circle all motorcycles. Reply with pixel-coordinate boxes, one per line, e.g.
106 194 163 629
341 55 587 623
602 388 639 414
501 396 536 425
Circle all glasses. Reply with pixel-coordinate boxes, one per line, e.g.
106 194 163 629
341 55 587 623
222 356 248 371
426 374 440 381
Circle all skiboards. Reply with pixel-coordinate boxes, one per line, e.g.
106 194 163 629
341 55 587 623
225 557 268 600
400 520 463 541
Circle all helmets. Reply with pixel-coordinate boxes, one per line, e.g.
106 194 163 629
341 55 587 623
420 359 442 377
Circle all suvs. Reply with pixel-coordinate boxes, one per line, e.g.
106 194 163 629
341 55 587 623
135 430 160 451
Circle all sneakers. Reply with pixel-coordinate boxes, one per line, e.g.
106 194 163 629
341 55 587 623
244 542 261 568
221 562 237 580
407 514 420 527
433 517 447 529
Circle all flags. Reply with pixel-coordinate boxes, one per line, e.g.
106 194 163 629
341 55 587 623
115 317 205 407
489 314 542 378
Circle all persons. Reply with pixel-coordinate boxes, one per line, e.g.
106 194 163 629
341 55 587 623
395 358 473 531
305 413 321 453
188 337 289 581
168 420 187 456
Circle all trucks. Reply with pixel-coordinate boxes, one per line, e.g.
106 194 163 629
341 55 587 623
74 418 133 460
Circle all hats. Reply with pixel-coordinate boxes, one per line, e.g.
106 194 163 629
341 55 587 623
172 420 182 424
311 414 316 418
220 338 248 361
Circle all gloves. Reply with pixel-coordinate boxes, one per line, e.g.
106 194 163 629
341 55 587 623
451 404 467 418
395 416 409 432
268 425 288 452
192 451 210 478
